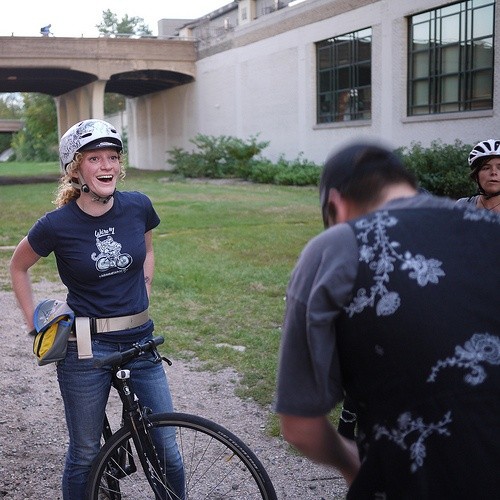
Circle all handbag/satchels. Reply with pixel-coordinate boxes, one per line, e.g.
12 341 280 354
32 298 74 367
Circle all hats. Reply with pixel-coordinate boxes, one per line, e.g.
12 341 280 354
319 143 406 230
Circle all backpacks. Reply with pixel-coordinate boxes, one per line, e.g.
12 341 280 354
338 369 500 500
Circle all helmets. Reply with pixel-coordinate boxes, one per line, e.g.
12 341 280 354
468 140 500 168
58 119 124 175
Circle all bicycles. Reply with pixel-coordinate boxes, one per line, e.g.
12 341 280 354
83 336 279 500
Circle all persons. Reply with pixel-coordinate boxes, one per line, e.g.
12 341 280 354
9 120 187 500
456 139 500 212
273 139 500 500
40 24 54 37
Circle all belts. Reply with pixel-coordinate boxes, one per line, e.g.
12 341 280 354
71 308 149 359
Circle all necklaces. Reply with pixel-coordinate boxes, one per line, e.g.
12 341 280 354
479 195 500 211
